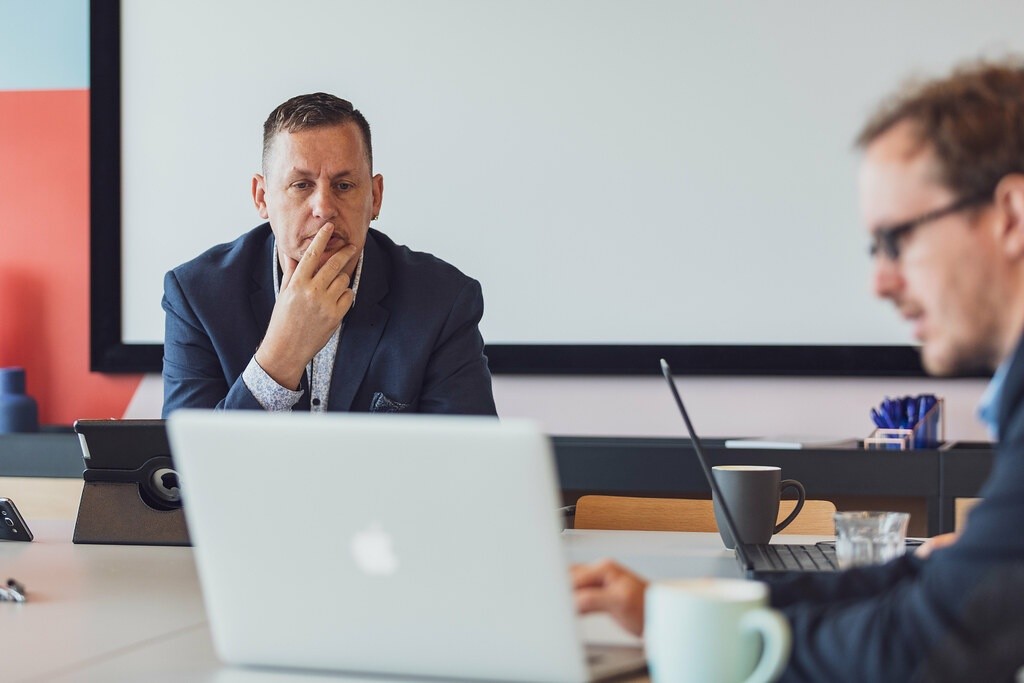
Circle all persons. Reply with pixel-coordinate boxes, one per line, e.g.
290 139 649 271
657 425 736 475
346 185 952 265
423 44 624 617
569 51 1024 683
159 92 500 417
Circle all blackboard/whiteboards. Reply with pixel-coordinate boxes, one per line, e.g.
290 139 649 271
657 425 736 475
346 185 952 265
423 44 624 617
89 2 1023 377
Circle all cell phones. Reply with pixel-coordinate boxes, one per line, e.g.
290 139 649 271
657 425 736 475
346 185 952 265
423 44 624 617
0 498 34 543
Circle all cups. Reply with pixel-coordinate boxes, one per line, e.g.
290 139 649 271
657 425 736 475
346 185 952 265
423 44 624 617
711 465 805 550
643 578 790 683
833 511 911 571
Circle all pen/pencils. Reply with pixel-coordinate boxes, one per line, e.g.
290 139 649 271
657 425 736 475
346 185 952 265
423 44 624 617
868 394 940 451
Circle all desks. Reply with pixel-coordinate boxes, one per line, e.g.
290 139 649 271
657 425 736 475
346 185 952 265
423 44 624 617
0 475 928 683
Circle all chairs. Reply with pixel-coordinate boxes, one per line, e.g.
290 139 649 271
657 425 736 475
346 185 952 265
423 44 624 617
575 494 837 538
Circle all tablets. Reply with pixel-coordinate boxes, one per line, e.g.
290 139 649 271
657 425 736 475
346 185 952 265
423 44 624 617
76 416 183 512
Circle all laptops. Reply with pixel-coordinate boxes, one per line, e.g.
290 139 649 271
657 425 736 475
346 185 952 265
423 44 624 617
168 407 648 683
660 359 929 576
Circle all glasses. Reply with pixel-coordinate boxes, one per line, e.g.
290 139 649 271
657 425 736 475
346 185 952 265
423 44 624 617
869 187 993 262
